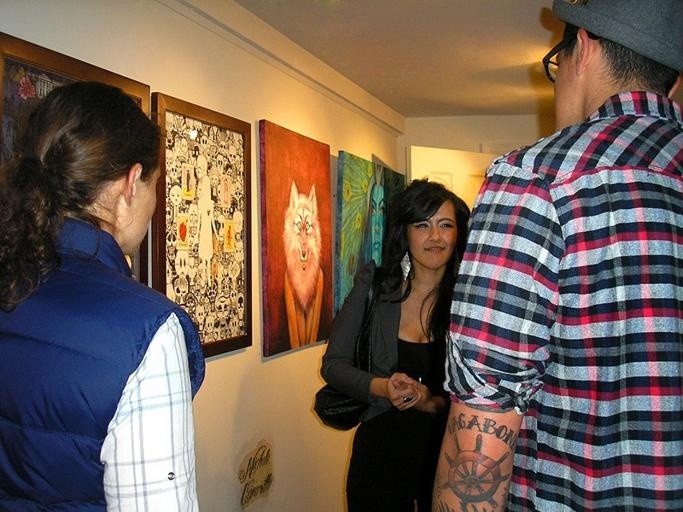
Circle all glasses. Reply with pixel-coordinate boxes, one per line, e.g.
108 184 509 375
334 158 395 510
541 31 598 83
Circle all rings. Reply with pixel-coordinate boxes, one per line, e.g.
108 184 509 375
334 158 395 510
402 395 412 402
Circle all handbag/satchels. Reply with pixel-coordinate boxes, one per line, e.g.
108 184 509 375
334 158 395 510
313 269 380 431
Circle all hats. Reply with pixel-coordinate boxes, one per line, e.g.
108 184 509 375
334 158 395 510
551 0 682 74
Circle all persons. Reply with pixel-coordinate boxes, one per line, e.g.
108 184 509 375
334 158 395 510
426 1 683 512
316 175 473 510
1 77 207 510
